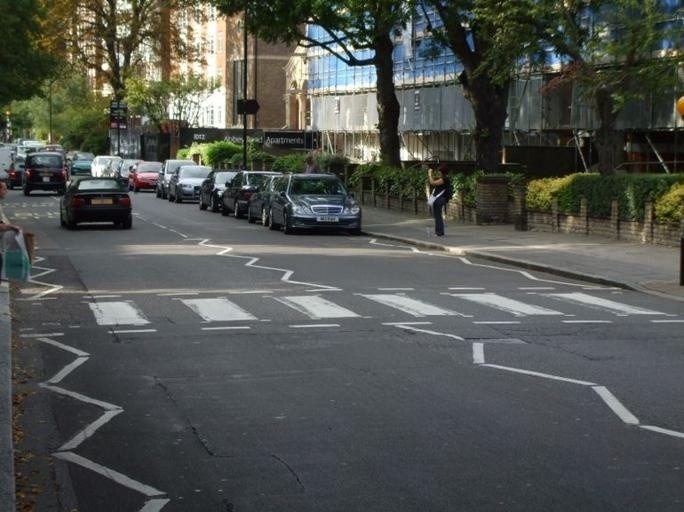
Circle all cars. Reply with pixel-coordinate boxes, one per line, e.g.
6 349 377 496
60 178 132 228
247 176 284 225
200 171 237 211
91 155 213 202
269 173 362 235
4 160 22 188
221 171 280 217
0 141 95 179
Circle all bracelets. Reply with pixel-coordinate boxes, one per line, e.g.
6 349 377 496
429 175 432 178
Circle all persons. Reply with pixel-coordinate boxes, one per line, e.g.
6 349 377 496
302 153 321 174
0 180 21 281
426 162 454 238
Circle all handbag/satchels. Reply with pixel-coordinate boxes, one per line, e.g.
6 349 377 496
23 233 35 264
427 194 436 206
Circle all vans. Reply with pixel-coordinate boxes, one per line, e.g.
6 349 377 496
23 153 66 194
